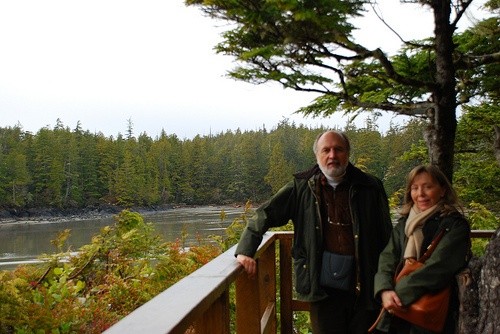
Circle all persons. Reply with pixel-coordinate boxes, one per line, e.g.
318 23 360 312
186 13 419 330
375 164 472 334
235 131 393 334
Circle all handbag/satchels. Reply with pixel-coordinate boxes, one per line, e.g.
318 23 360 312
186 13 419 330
388 258 450 332
319 251 354 291
